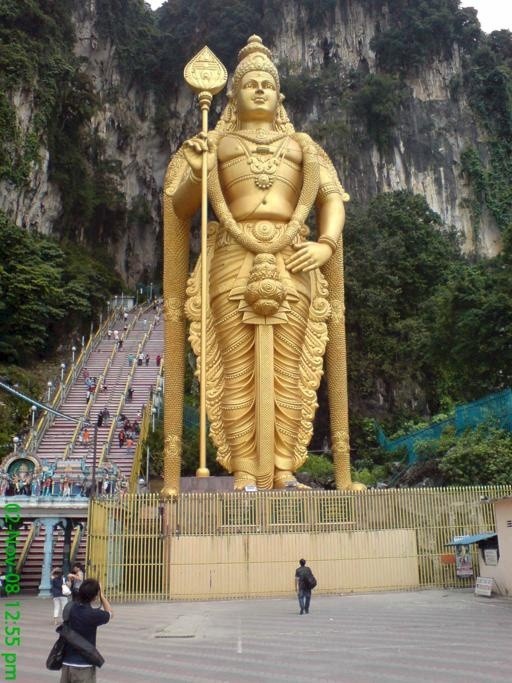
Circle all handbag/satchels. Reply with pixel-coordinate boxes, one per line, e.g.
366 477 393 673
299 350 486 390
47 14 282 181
57 624 104 667
62 584 72 596
304 573 317 590
45 638 67 672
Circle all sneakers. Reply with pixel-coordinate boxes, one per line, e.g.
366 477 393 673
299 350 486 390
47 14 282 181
300 607 308 614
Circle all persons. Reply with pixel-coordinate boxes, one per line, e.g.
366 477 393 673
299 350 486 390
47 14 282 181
67 562 85 601
158 35 370 505
41 467 150 497
50 567 72 626
60 575 114 682
78 287 164 455
294 558 314 615
